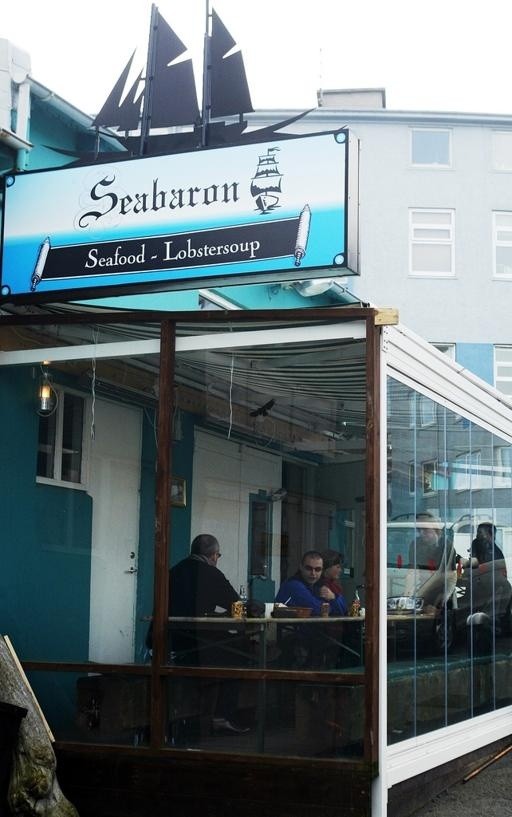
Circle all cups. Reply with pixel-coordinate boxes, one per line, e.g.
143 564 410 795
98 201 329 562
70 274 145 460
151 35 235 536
264 601 274 618
320 602 329 617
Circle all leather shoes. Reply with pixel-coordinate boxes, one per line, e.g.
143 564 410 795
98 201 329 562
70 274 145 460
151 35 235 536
211 715 252 737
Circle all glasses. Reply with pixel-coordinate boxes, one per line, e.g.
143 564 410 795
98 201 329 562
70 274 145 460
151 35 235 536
301 564 324 573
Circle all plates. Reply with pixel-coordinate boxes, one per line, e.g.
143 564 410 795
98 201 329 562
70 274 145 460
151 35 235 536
387 608 424 614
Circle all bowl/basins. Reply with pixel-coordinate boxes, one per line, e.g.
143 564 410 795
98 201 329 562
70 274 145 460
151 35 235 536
387 595 424 609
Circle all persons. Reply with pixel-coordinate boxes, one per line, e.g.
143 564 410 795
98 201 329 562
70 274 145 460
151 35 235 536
474 522 508 581
430 537 512 653
269 550 348 668
319 551 345 597
146 532 253 736
407 511 458 572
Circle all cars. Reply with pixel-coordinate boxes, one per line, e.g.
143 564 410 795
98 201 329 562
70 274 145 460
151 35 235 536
374 512 509 656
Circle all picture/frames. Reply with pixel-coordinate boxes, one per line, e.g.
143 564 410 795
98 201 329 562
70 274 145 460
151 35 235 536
407 445 512 500
169 475 187 507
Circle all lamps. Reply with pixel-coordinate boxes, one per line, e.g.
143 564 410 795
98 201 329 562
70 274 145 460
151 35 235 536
33 359 60 419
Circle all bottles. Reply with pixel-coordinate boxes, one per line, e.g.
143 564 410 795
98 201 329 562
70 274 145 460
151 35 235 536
352 588 360 616
239 585 248 617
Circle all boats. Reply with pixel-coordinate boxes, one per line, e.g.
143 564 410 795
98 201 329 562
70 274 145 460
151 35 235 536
250 146 285 215
